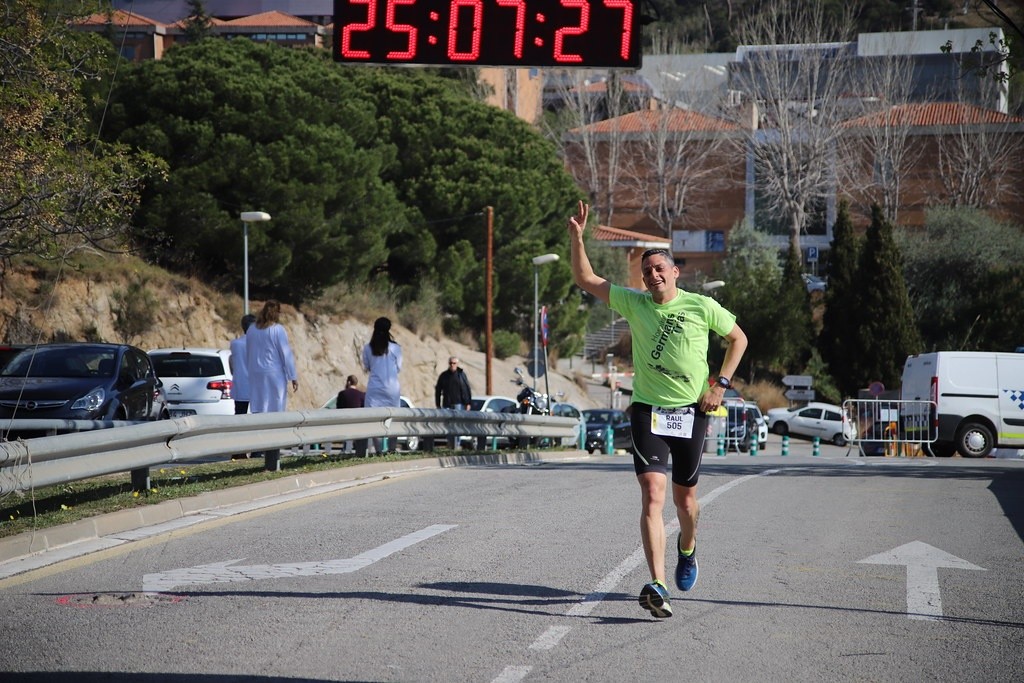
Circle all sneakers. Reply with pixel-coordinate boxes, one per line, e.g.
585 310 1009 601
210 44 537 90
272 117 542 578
675 532 698 592
639 579 673 618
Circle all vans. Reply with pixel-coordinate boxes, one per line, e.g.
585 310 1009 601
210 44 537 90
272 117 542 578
898 351 1024 458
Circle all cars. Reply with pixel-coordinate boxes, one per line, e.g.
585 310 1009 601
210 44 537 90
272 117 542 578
0 342 171 443
145 347 251 418
725 405 759 453
763 402 856 447
321 394 419 455
581 409 632 454
724 401 770 449
859 422 923 457
548 402 586 449
419 395 520 450
803 272 826 293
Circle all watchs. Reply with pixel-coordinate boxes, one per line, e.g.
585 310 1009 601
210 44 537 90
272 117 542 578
716 377 733 389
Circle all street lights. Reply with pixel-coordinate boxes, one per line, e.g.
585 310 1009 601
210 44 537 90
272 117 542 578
532 253 560 390
241 211 271 316
702 279 726 374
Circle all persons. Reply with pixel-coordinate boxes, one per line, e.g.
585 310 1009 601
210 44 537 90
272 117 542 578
230 314 256 460
246 300 299 457
336 375 366 409
435 356 472 448
569 200 748 619
363 317 402 455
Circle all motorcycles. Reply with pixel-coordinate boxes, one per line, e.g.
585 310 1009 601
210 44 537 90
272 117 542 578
510 368 565 449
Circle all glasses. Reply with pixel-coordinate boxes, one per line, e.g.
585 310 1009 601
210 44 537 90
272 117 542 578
449 362 457 364
